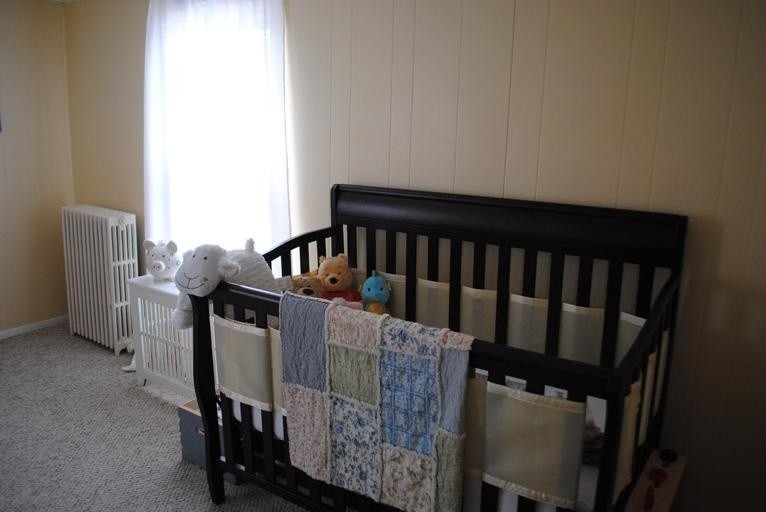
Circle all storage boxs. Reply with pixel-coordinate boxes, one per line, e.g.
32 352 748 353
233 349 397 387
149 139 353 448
177 390 248 486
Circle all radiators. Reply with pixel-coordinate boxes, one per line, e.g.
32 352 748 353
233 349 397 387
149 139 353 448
60 204 139 356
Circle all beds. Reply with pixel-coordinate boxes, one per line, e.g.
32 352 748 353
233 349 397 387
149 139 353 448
188 184 688 511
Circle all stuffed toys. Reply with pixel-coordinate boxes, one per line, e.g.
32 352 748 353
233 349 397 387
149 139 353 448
171 237 280 330
143 240 182 283
289 275 324 298
317 254 361 302
359 270 393 315
